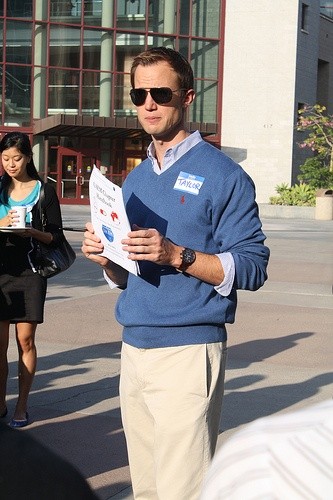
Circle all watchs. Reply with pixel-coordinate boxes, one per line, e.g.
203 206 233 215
176 246 197 273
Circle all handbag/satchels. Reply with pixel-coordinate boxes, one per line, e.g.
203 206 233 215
29 183 76 278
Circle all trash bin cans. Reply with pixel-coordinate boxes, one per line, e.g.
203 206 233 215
315 189 333 221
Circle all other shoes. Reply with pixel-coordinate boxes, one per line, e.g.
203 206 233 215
8 411 28 427
0 406 8 417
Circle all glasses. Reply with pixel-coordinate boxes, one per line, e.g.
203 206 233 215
129 87 180 106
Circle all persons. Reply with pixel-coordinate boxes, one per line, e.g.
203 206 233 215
0 132 64 429
81 48 269 500
